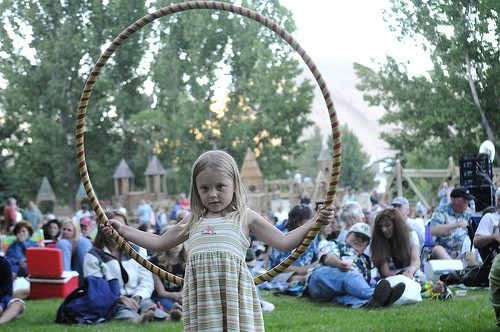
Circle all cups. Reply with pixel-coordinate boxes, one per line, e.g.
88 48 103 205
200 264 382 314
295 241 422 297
340 256 354 268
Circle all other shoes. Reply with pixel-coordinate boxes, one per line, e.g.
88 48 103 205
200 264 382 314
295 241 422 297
261 301 275 311
171 303 182 320
384 282 405 307
365 279 391 308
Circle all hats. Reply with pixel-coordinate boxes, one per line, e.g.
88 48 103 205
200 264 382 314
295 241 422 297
289 206 310 216
391 196 409 205
80 217 93 227
346 222 372 238
451 188 476 200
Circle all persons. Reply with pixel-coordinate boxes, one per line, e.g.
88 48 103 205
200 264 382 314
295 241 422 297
307 223 405 309
319 196 425 257
75 206 90 222
5 221 40 297
461 206 496 267
4 197 42 232
0 255 25 324
342 190 358 203
135 193 191 232
150 225 190 310
44 218 94 281
415 206 436 246
83 211 183 323
245 204 319 298
99 150 336 332
371 208 453 300
430 187 474 275
438 181 450 204
475 186 500 327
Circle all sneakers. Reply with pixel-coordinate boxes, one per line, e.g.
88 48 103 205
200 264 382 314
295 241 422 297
127 309 154 324
141 307 170 320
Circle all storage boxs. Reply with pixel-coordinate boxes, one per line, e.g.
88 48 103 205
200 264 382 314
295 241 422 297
27 247 79 299
425 257 463 281
